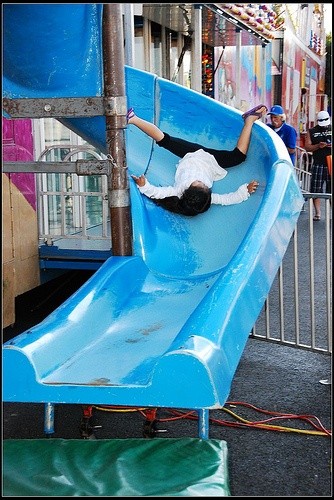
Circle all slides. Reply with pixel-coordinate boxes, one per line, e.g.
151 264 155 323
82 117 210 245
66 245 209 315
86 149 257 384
0 2 306 412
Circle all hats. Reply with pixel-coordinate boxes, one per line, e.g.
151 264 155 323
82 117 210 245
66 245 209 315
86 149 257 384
317 111 330 126
268 105 284 115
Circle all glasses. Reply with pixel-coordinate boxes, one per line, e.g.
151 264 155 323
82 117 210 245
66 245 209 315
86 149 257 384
318 117 329 122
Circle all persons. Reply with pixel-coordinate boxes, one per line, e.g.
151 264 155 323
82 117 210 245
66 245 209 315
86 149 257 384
304 111 332 220
266 105 297 167
125 104 268 216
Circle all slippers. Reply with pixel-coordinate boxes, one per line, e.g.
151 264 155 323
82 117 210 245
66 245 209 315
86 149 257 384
241 104 268 121
126 107 136 125
314 216 321 222
